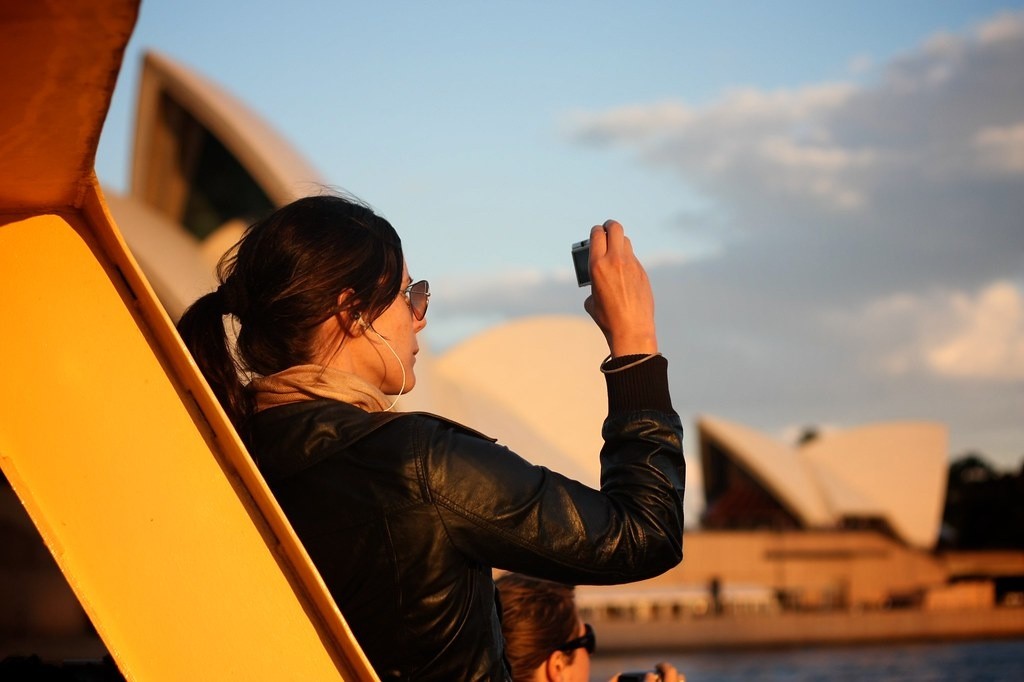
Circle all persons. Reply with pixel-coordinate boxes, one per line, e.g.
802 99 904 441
176 195 686 682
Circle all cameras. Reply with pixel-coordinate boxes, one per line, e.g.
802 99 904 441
571 239 591 287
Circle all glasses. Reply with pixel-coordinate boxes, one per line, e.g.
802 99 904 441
548 623 596 654
398 280 430 320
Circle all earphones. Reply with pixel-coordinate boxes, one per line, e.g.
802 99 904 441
352 312 367 328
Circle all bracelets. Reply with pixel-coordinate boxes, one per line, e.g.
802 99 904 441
600 352 663 374
